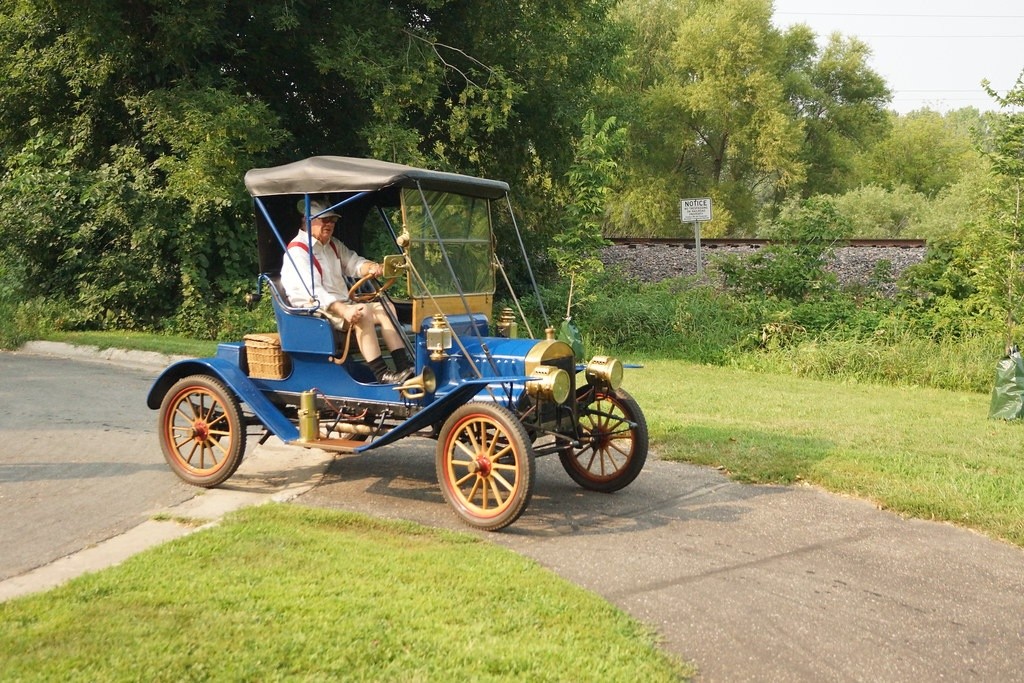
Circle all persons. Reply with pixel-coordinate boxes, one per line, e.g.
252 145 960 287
279 199 415 385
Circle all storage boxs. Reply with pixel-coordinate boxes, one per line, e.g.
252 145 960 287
243 332 291 380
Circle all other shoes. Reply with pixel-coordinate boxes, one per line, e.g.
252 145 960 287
410 367 415 374
381 365 413 384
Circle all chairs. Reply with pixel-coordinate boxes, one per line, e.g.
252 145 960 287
260 272 416 357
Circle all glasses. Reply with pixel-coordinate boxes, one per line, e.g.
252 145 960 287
322 216 337 223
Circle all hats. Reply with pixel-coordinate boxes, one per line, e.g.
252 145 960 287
297 194 342 218
